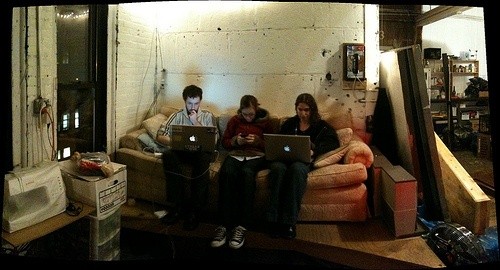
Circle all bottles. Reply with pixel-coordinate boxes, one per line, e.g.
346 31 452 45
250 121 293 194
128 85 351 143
453 63 472 72
452 86 456 96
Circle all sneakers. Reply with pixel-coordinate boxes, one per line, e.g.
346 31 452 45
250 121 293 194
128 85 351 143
212 226 227 246
229 226 247 248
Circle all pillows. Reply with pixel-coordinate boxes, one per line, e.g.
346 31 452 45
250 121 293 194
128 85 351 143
313 128 353 167
138 113 171 153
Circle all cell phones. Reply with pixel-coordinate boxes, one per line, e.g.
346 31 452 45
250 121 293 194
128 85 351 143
240 134 248 139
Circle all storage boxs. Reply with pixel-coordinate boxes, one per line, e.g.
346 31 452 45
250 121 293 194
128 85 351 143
60 159 128 215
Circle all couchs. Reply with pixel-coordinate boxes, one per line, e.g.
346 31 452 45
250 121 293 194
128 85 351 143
115 104 375 224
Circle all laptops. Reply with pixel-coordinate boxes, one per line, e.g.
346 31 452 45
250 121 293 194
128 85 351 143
170 124 216 153
263 134 315 163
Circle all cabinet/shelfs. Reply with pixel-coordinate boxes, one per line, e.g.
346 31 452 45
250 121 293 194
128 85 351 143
422 57 480 149
0 195 121 264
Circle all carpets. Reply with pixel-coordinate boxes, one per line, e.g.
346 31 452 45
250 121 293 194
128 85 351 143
0 217 434 270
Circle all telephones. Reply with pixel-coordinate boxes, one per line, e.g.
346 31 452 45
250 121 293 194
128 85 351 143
343 43 365 81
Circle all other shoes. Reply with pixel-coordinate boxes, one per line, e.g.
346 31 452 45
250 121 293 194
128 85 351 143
282 222 296 240
161 210 181 225
269 224 281 239
183 211 199 231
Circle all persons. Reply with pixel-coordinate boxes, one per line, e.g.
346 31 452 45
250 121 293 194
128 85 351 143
157 85 221 231
267 93 339 239
211 95 277 249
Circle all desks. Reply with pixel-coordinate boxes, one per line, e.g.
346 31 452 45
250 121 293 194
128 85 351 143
455 107 489 149
